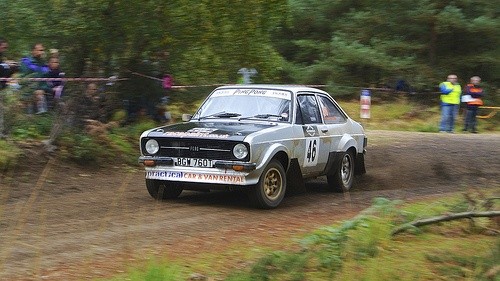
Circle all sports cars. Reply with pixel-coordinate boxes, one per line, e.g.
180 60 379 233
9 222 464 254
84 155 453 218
138 83 368 210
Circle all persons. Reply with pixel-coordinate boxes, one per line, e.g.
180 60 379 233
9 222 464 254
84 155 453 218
0 40 175 137
438 74 462 133
237 62 258 84
463 76 484 132
